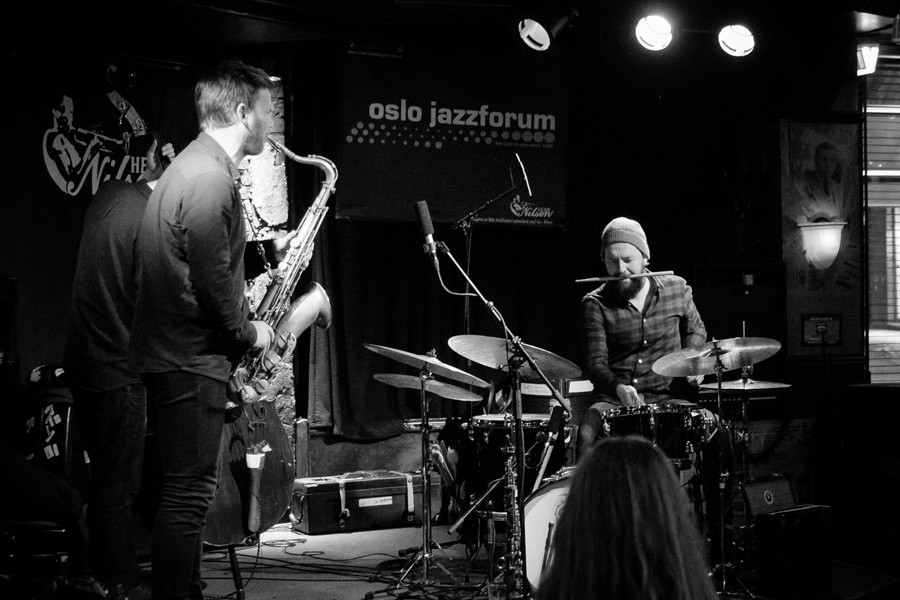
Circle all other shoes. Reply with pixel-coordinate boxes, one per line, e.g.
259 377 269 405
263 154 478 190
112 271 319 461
113 583 152 600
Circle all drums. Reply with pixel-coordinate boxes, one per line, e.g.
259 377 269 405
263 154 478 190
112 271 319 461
520 465 581 594
603 402 695 470
22 364 91 481
468 412 568 520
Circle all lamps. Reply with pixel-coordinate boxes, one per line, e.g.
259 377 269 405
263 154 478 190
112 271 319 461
516 0 762 58
797 222 848 273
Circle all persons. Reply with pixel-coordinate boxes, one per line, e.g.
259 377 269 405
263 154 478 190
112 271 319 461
129 60 276 600
539 437 719 600
75 140 176 600
575 217 709 460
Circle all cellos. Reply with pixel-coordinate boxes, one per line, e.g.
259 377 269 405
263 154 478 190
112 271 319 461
203 398 297 549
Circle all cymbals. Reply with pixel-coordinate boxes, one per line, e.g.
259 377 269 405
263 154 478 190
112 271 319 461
363 341 492 388
371 373 484 403
448 334 582 382
651 336 782 379
697 378 793 391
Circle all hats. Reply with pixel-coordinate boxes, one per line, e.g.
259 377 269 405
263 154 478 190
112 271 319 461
601 217 650 261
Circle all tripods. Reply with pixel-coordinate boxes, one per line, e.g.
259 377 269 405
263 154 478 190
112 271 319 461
365 364 509 600
705 349 758 600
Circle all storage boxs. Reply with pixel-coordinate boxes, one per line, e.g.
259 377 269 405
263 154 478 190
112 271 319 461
290 470 442 534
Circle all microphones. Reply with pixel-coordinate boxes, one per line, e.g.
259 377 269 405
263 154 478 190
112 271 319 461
414 201 439 269
540 405 564 462
514 153 533 197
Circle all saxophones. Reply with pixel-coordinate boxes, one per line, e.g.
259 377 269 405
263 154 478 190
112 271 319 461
226 137 339 404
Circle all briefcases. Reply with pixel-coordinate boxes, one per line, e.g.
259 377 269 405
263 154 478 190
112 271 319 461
290 469 441 534
